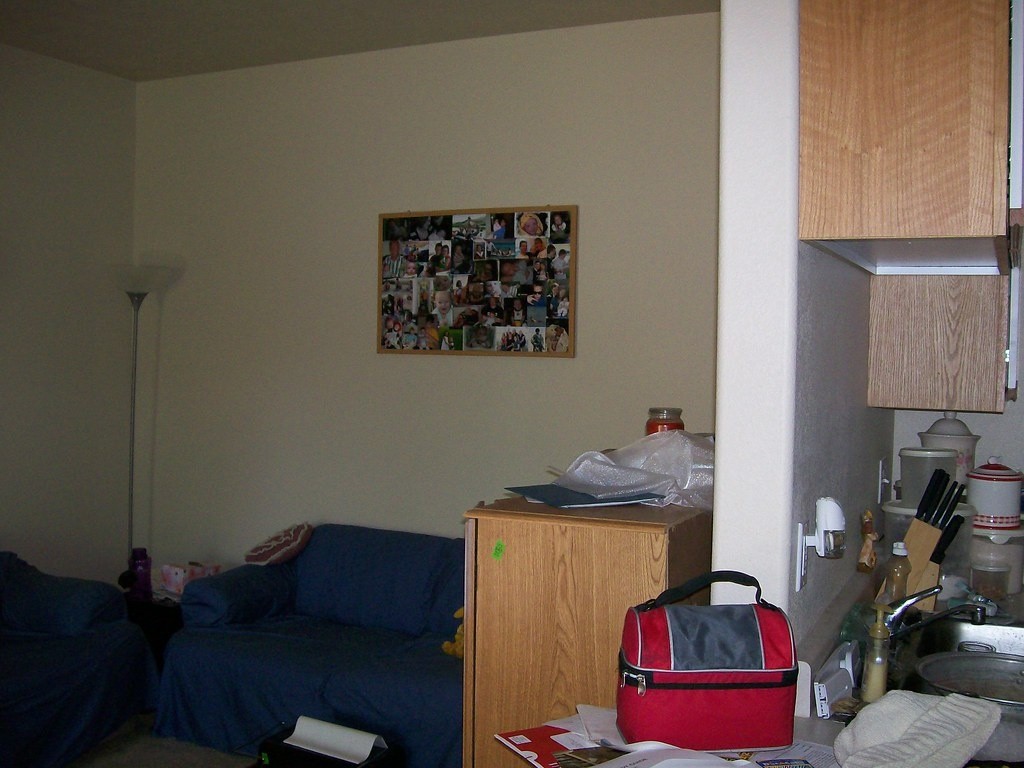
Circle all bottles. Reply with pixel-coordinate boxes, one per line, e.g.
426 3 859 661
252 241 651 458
128 546 152 599
884 541 915 606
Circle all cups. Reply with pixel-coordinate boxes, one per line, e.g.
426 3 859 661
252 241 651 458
915 411 980 496
966 454 1023 530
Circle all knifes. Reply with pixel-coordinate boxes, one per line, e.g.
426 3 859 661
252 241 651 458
875 466 969 611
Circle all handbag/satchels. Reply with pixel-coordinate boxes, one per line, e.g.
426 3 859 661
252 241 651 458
616 570 799 752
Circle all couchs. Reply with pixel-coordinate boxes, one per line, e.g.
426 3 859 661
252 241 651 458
0 550 136 768
153 524 462 768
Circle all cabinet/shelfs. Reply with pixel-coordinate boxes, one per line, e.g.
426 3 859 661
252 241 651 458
796 0 1010 275
462 495 713 768
866 268 1019 413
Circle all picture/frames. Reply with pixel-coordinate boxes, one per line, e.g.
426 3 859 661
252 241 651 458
375 205 579 357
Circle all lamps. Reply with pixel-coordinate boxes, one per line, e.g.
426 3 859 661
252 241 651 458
118 272 148 587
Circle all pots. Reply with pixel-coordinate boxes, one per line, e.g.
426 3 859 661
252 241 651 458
914 640 1024 765
